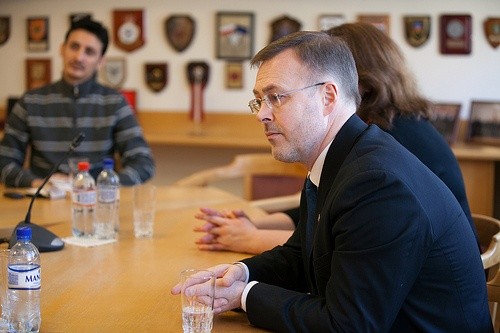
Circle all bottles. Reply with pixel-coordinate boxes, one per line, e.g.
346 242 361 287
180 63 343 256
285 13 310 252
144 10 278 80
6 225 43 333
71 161 96 238
94 158 121 240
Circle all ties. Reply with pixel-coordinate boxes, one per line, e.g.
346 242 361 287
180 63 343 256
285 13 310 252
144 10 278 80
305 180 318 258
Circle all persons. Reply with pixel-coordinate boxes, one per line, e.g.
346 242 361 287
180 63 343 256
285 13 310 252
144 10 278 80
173 33 495 333
193 25 488 278
0 17 155 190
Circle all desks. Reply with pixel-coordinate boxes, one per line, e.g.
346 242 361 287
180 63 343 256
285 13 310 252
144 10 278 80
452 141 500 216
0 179 273 333
138 113 271 164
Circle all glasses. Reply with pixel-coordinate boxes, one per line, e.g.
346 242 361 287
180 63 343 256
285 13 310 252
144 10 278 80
248 82 331 114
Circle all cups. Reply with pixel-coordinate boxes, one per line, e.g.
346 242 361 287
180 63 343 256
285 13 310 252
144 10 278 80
131 184 156 238
0 249 10 308
181 268 217 332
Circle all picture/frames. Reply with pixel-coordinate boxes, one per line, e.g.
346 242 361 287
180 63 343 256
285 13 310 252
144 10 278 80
215 11 254 61
440 14 472 53
467 100 500 146
429 104 463 145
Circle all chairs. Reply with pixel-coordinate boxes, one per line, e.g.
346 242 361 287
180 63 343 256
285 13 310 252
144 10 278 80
180 151 311 215
471 213 500 333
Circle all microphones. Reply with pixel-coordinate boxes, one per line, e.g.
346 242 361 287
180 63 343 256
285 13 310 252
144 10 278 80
8 133 86 252
